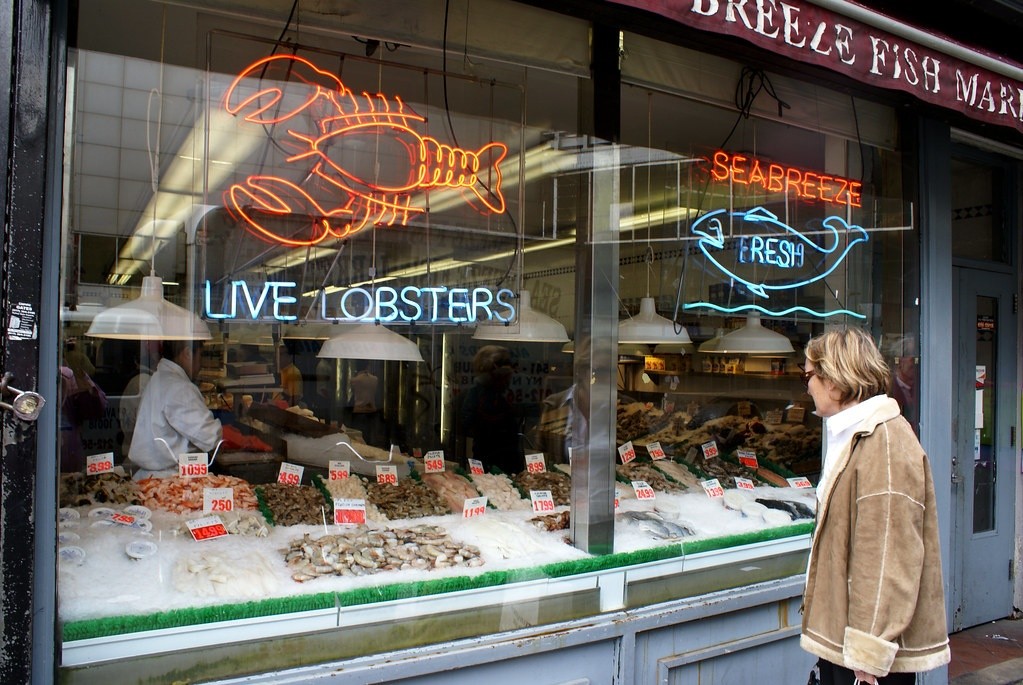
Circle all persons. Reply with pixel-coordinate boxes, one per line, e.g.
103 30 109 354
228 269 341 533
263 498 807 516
126 340 273 482
528 334 649 470
279 344 544 483
57 318 304 490
799 326 952 685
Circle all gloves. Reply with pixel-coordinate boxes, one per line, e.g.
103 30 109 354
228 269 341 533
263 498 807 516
221 424 272 453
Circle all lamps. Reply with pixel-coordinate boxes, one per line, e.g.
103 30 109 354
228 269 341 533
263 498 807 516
612 88 692 357
695 125 795 357
84 43 424 363
471 59 571 343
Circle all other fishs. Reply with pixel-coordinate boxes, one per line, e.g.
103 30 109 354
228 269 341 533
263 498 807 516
754 498 816 521
615 510 696 540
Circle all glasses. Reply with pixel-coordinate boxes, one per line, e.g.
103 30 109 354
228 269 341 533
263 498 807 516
798 369 816 390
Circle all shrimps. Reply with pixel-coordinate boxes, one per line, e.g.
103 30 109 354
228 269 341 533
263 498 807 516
278 524 485 582
138 472 259 515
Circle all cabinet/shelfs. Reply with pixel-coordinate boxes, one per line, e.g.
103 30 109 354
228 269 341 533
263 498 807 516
620 304 824 379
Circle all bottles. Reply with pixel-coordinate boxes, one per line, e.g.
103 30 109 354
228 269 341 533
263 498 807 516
619 280 803 375
